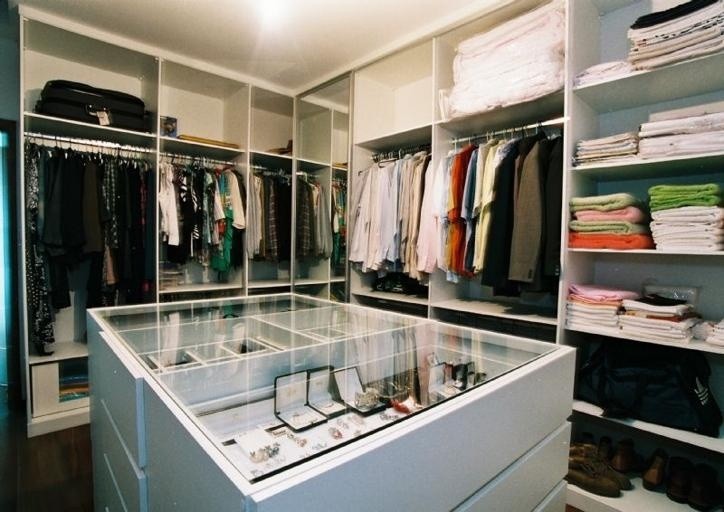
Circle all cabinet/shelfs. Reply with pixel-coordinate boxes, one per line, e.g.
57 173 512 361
9 1 292 439
85 292 577 512
352 1 724 512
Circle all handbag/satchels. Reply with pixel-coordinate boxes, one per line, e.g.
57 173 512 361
574 335 724 440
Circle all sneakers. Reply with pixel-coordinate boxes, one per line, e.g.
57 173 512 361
566 431 724 511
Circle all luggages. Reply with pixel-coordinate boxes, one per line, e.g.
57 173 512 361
34 78 145 131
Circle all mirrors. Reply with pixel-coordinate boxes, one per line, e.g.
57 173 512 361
293 66 351 305
